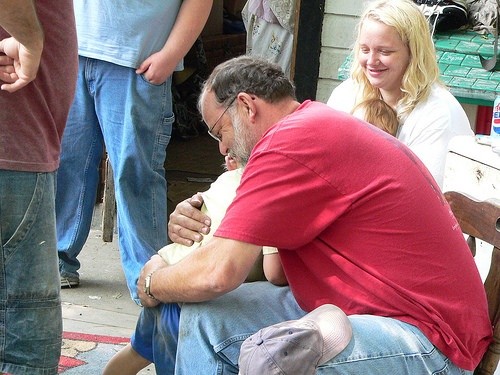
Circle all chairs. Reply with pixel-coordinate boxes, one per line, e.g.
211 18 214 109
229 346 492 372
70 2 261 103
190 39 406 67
444 190 500 375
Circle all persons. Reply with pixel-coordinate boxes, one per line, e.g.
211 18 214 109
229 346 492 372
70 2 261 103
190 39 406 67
137 56 493 375
102 154 291 375
53 0 213 306
327 0 474 192
0 0 78 375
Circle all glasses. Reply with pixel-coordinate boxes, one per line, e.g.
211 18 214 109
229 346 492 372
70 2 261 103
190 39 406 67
207 90 246 143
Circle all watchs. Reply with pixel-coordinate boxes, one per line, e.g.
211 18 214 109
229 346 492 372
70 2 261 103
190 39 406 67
143 270 156 299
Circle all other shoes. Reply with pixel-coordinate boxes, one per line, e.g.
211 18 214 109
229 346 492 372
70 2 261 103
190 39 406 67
60 273 79 287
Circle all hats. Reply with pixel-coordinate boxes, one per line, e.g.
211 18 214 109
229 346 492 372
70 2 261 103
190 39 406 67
238 303 353 375
